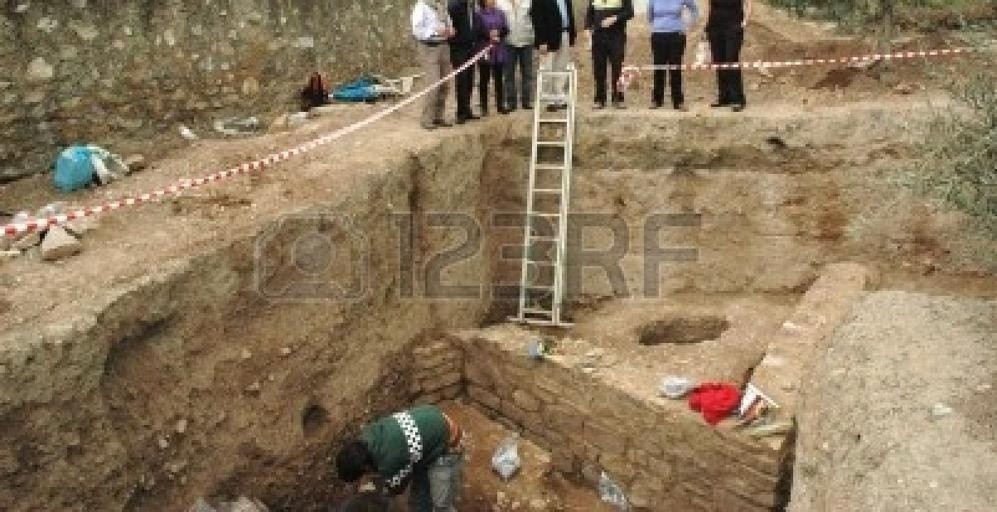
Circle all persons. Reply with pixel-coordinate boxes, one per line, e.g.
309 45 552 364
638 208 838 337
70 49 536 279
583 0 635 111
701 1 753 112
472 0 510 118
447 0 486 125
532 0 580 113
494 0 534 112
335 404 464 511
409 1 457 130
647 0 701 113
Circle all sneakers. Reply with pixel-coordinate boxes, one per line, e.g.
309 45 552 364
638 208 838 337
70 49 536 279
546 104 567 112
425 105 532 130
592 101 626 110
649 102 690 111
710 102 744 112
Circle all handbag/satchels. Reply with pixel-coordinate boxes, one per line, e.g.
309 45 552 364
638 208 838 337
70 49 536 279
690 382 743 425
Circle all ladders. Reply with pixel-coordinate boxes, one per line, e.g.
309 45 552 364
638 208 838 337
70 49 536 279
506 70 576 327
